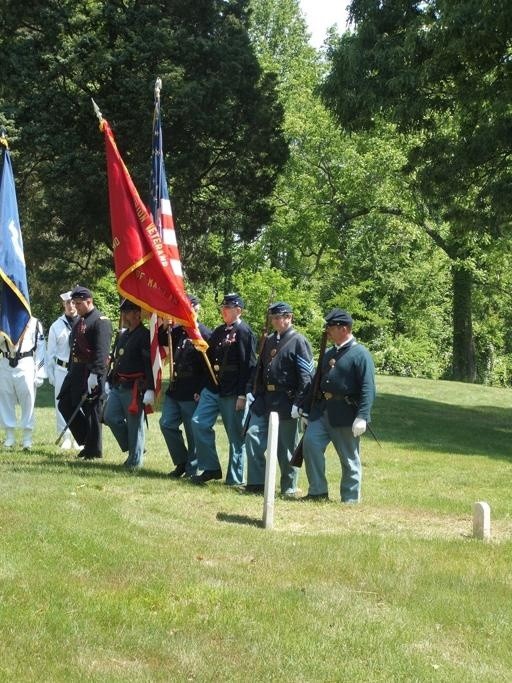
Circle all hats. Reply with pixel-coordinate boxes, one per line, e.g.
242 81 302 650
326 308 352 326
188 294 197 306
269 302 292 313
220 294 243 308
60 287 92 301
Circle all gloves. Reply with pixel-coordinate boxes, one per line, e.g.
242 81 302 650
143 389 154 406
352 418 367 438
87 374 98 394
35 378 44 387
246 393 255 406
291 405 308 432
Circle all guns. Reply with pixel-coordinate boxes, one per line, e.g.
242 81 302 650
100 292 125 424
241 286 275 441
287 330 327 467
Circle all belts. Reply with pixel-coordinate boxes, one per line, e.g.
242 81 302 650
2 352 33 357
317 391 344 401
57 359 68 367
214 364 239 371
71 356 86 363
264 384 287 392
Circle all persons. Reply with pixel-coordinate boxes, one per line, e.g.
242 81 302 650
102 298 156 468
158 294 214 478
44 289 86 451
295 307 376 504
0 315 46 450
56 286 116 459
243 301 315 495
189 292 258 486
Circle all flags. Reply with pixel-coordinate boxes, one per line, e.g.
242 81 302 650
136 105 184 391
0 139 33 347
98 118 209 355
